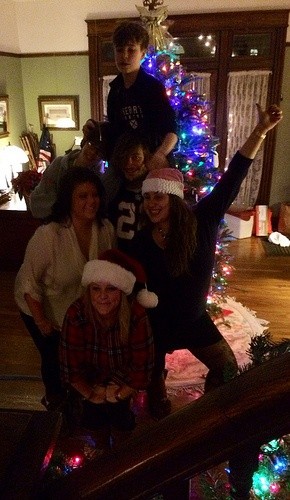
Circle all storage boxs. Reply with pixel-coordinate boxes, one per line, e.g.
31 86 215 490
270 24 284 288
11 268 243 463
224 213 254 239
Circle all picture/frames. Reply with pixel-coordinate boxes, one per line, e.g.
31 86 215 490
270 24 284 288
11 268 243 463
38 96 80 131
0 94 9 139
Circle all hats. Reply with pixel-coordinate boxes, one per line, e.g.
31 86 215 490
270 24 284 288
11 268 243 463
81 249 159 307
142 169 184 198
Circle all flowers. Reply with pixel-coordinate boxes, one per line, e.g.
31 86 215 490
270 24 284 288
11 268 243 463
10 170 42 211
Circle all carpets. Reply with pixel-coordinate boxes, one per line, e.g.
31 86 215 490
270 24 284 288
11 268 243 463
261 237 290 256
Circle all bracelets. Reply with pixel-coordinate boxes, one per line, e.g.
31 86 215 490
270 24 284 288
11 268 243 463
114 387 122 402
253 129 266 139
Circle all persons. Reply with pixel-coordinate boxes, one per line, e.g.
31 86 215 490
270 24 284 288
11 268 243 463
125 103 282 414
29 22 178 250
60 249 158 443
15 164 119 412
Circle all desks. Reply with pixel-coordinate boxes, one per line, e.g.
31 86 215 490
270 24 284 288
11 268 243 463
0 190 42 263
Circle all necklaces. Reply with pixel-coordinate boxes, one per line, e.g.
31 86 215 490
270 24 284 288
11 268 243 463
156 225 167 240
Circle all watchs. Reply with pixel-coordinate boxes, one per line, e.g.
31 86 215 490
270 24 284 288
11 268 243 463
81 389 94 401
